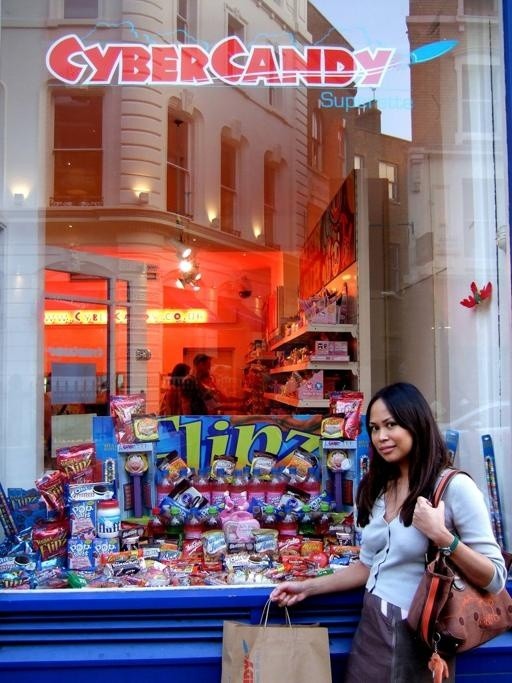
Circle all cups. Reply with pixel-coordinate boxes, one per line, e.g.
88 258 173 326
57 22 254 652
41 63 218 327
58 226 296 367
336 531 353 547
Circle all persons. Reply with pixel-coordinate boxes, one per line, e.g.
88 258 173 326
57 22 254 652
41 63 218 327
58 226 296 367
159 363 190 415
180 353 217 416
202 368 245 415
266 380 508 683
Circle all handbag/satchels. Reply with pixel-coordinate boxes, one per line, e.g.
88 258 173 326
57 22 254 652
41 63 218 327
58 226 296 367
219 595 335 683
407 545 511 661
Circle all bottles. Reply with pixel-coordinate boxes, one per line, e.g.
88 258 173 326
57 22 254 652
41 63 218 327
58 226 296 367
95 497 334 552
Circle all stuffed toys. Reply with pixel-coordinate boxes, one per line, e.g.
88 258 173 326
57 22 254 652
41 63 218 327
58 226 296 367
427 653 449 683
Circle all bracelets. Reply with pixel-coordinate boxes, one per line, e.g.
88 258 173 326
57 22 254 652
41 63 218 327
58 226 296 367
438 532 459 558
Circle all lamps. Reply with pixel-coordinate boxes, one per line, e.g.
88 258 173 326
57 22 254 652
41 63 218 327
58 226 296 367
171 236 202 291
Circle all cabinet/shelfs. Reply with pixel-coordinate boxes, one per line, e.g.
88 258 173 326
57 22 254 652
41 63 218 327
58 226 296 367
244 323 360 409
